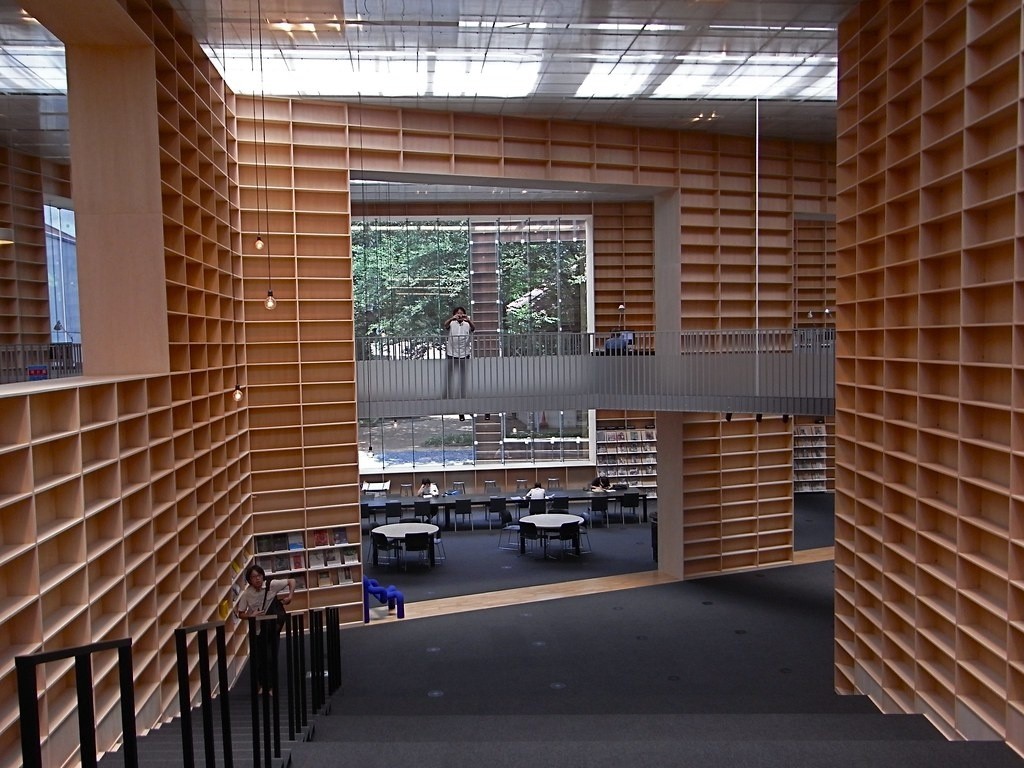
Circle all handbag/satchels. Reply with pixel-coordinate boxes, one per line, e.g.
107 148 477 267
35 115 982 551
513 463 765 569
261 577 287 636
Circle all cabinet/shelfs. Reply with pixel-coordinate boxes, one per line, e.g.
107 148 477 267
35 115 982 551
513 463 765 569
2 1 1023 768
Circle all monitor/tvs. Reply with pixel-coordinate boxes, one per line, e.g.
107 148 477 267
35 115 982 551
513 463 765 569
619 330 635 345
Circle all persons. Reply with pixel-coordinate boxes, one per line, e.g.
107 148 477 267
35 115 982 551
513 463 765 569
590 476 610 518
440 306 475 400
604 328 626 356
526 481 546 513
416 477 440 523
238 564 297 697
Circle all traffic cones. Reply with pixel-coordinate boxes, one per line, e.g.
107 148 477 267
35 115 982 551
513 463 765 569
538 411 548 429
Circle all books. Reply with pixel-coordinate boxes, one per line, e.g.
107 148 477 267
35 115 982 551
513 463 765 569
218 526 360 619
595 430 657 486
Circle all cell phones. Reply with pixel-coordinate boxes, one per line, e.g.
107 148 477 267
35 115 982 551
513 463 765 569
459 315 467 319
